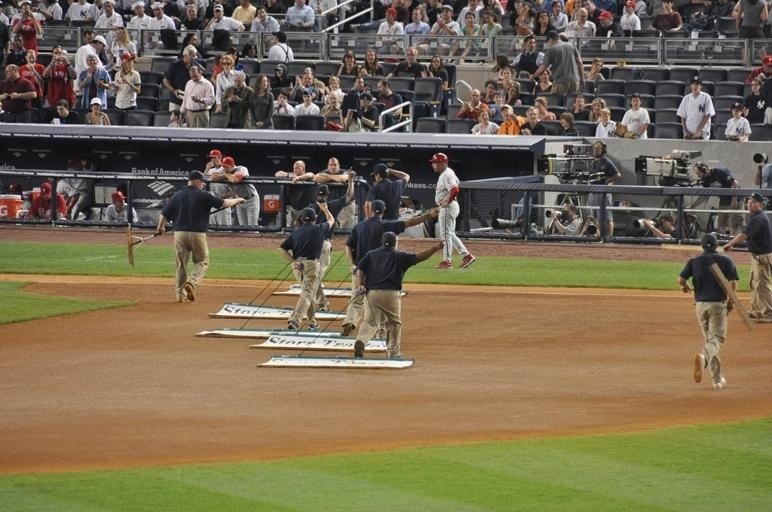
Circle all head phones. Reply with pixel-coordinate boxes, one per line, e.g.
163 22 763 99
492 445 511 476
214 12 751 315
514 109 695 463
695 162 708 171
602 144 607 153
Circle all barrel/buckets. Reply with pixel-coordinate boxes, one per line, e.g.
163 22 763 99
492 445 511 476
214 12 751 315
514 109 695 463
263 194 280 213
0 194 24 219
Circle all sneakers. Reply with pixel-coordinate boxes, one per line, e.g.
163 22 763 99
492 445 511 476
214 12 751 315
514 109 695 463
690 351 708 384
176 294 192 303
352 340 367 360
459 252 476 270
756 313 772 324
287 320 301 331
711 374 729 391
746 308 761 319
318 299 330 312
341 322 354 336
433 260 454 270
183 282 198 302
306 322 321 332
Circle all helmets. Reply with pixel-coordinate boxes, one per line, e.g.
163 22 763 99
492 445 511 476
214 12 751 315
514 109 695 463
426 152 450 166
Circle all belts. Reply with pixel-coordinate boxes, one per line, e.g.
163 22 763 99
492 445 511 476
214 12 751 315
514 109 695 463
751 250 772 255
294 256 319 261
436 204 442 207
367 287 400 291
696 299 723 303
324 236 331 241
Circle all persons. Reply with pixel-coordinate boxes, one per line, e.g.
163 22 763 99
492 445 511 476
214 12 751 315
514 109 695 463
156 170 246 303
723 192 772 323
278 202 335 330
354 231 446 358
427 153 476 269
678 233 740 390
294 165 356 310
341 200 440 336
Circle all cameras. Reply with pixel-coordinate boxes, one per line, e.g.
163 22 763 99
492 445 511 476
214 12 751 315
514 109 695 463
55 56 64 61
24 10 30 15
753 153 768 165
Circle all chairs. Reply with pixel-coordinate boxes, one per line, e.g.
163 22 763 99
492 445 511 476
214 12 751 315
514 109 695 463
159 86 171 97
156 97 172 111
154 111 172 126
42 20 68 40
101 109 125 125
151 58 177 72
592 16 772 51
139 71 164 83
37 53 52 66
137 96 157 111
125 110 154 125
209 59 342 87
342 62 457 100
209 112 227 129
567 67 772 140
272 114 342 131
609 108 628 122
107 96 116 109
517 79 566 135
71 20 96 40
414 100 477 134
138 83 158 96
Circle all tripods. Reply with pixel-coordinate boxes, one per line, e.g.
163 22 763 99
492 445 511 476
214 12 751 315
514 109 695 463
643 195 689 244
545 179 583 235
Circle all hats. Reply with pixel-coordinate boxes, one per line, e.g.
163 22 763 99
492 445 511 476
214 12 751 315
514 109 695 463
369 199 388 214
186 169 213 184
315 183 332 196
207 149 223 159
300 207 318 224
701 234 718 247
0 0 772 113
367 162 392 178
39 182 51 199
745 190 764 204
217 156 236 167
380 231 398 248
109 190 127 201
